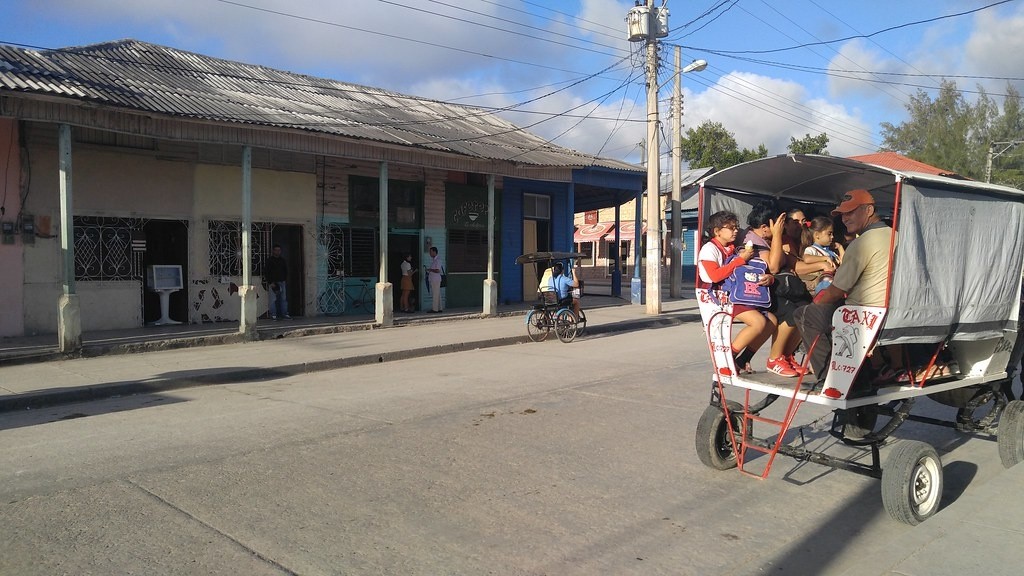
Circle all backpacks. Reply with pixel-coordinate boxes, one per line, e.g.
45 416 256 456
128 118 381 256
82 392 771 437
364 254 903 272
708 254 771 311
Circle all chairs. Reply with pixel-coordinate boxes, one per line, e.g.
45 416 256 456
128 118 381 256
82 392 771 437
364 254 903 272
536 291 572 310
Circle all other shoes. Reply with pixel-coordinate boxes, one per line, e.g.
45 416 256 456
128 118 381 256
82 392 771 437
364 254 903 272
401 309 415 313
733 358 756 376
801 378 826 392
578 317 584 321
873 364 910 383
427 309 442 313
271 317 277 322
282 314 293 320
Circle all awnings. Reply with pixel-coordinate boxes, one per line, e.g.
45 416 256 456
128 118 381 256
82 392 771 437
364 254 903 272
573 222 647 242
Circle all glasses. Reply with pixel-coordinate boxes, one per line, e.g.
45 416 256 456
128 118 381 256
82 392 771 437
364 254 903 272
722 225 740 231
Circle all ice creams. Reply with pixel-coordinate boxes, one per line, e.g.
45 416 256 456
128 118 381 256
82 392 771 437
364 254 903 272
744 240 753 249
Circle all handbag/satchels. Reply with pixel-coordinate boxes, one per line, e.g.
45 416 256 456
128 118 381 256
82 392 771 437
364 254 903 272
813 271 835 304
440 275 447 287
261 276 279 292
767 265 806 299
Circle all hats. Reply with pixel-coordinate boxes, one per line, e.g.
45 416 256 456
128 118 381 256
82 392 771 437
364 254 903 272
831 189 875 216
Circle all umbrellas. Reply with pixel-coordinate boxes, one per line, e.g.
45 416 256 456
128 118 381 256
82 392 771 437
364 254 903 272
425 247 442 313
423 265 429 293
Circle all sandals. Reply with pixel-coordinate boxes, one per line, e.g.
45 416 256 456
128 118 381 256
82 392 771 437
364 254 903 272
913 359 962 383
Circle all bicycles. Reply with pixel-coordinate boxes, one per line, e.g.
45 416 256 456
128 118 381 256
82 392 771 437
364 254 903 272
319 279 376 317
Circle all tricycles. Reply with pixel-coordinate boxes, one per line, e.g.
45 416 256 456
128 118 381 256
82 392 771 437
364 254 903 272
516 251 587 344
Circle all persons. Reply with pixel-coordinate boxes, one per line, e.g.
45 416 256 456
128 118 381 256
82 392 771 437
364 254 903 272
537 261 579 324
400 252 418 313
264 246 292 322
697 188 961 399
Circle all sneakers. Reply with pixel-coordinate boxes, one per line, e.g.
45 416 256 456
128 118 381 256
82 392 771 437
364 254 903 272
784 352 807 375
766 355 799 378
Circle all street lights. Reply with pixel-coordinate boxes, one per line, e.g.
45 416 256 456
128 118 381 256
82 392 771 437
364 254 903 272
645 58 708 316
